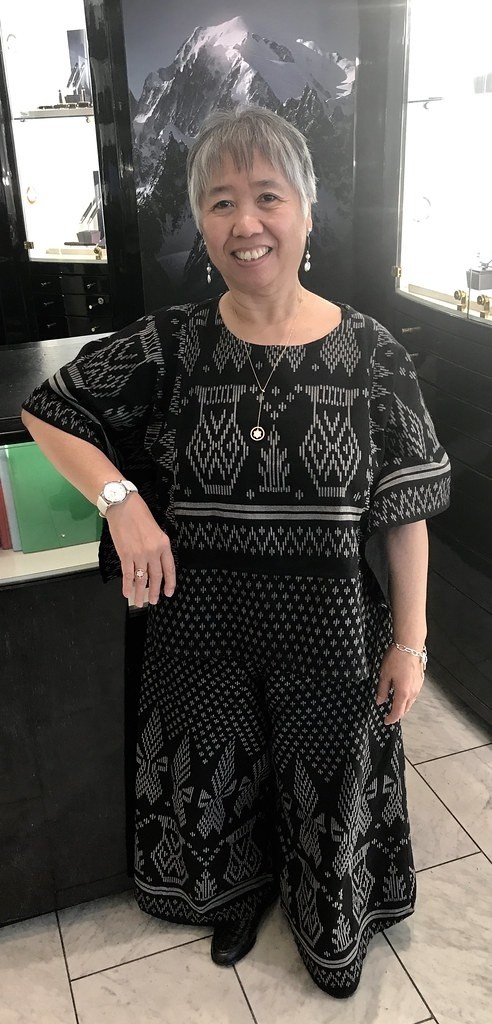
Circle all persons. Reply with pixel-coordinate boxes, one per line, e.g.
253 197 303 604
23 107 451 997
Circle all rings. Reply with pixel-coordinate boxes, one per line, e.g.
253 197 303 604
134 569 147 579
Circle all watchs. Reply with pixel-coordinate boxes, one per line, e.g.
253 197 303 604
96 479 139 518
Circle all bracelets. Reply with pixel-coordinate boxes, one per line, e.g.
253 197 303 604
388 640 428 671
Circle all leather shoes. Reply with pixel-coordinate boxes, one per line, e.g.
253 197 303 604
211 919 259 967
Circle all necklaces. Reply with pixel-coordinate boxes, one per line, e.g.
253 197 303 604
231 303 300 442
